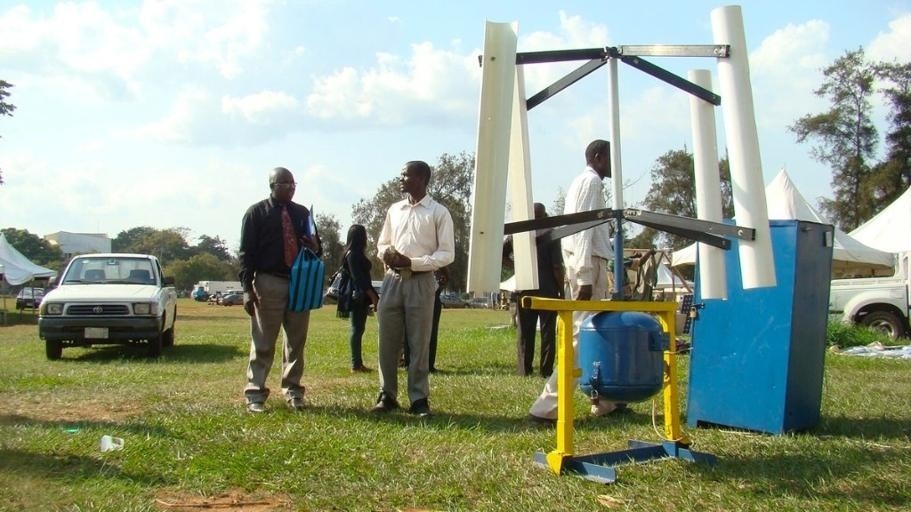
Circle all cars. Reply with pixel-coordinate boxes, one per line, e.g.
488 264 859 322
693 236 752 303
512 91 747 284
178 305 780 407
439 293 468 309
15 286 44 310
191 278 244 308
469 296 492 309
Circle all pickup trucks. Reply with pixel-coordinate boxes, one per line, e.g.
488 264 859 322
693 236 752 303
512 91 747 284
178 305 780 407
35 249 178 358
828 254 910 347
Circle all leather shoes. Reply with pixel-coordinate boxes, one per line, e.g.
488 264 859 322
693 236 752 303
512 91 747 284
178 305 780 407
247 403 269 415
605 407 633 419
289 398 307 412
410 399 432 420
371 400 397 414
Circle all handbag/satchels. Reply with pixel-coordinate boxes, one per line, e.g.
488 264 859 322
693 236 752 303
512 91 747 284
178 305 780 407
324 268 365 311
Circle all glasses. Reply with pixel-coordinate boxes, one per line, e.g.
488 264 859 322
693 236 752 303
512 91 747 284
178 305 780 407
273 182 298 189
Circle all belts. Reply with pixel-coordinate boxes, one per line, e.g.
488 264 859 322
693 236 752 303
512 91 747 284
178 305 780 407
262 270 289 279
390 267 430 279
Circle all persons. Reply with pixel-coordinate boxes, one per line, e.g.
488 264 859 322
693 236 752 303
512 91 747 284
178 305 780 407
236 167 323 416
370 161 455 419
336 225 378 372
607 245 654 301
502 203 565 377
530 140 635 422
403 267 451 373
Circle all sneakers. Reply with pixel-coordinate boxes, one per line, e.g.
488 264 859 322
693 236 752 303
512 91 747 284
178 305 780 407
352 366 371 374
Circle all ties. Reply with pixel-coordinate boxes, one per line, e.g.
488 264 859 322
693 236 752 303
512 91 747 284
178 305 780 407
281 204 299 268
